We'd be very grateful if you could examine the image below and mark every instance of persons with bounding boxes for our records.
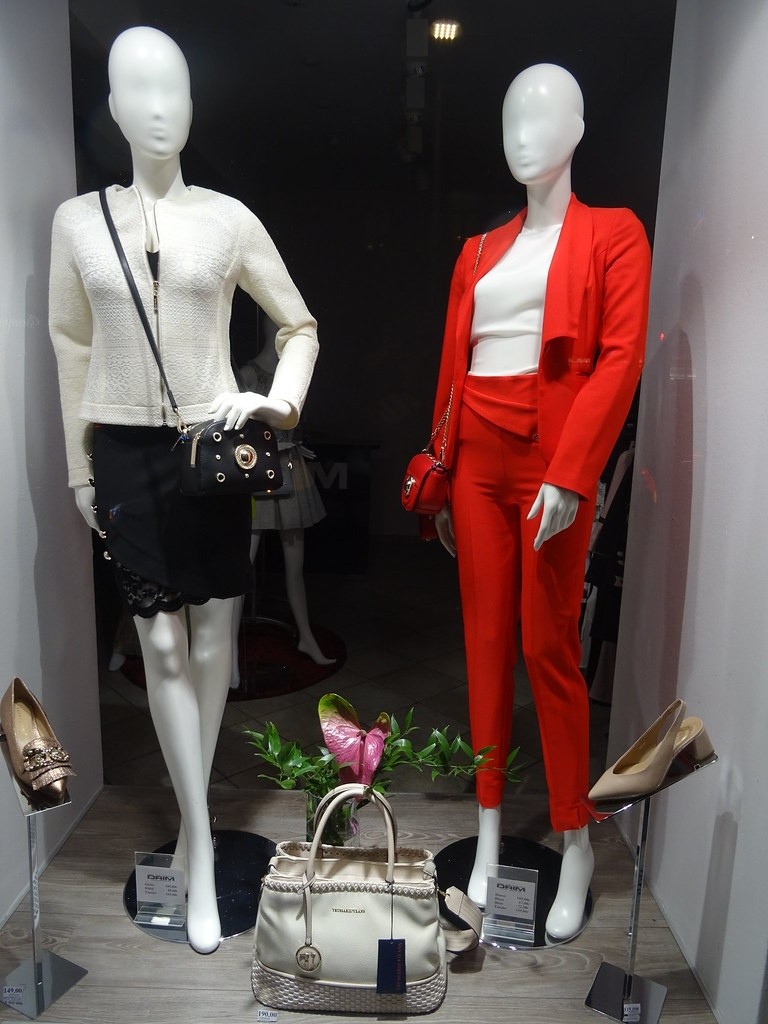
[226,313,337,688]
[48,25,322,954]
[432,64,650,939]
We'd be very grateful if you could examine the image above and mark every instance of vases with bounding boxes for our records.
[305,791,360,847]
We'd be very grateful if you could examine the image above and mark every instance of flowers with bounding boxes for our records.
[241,692,527,846]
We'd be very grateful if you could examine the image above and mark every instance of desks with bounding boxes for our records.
[259,436,382,576]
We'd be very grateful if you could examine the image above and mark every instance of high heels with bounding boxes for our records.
[589,695,714,801]
[0,677,78,809]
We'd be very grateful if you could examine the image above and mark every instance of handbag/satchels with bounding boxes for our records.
[398,234,488,520]
[94,188,287,507]
[249,780,447,1015]
